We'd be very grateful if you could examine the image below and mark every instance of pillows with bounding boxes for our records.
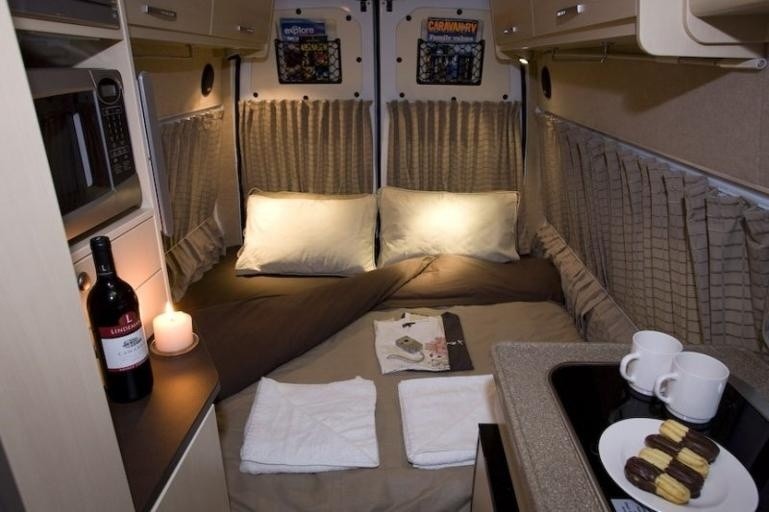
[376,185,521,269]
[235,190,379,278]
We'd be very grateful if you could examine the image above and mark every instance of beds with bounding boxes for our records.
[175,245,586,512]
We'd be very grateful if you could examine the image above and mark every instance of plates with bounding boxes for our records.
[597,417,758,511]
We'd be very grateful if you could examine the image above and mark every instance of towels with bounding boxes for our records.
[397,374,494,470]
[239,375,380,475]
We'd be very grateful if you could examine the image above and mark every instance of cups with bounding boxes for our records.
[620,329,730,424]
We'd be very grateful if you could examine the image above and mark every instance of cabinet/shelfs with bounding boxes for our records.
[74,216,168,387]
[470,424,519,512]
[125,0,273,50]
[490,0,639,52]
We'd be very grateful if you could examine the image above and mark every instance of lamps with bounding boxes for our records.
[153,301,194,353]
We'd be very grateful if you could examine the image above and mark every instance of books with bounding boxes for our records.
[424,14,480,84]
[275,15,332,84]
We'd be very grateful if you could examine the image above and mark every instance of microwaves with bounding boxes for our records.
[24,66,143,243]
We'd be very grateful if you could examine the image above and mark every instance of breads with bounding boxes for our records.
[626,418,720,505]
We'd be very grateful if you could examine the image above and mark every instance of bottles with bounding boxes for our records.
[83,233,155,401]
